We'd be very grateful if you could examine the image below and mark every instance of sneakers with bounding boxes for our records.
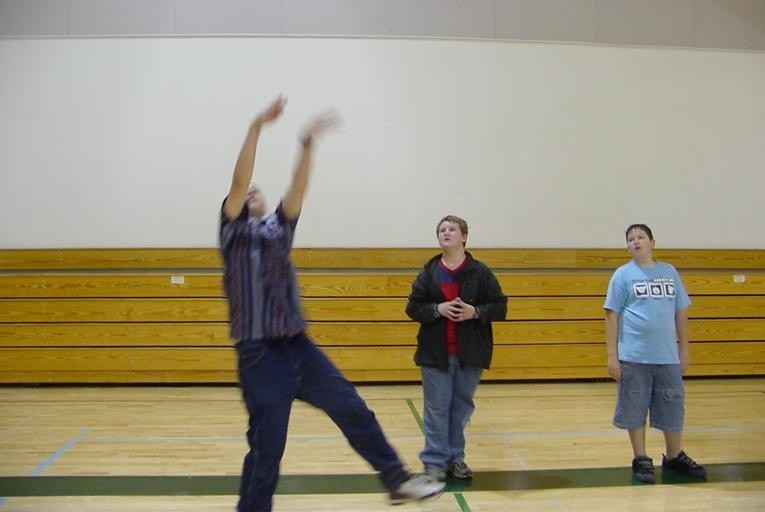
[389,475,446,506]
[662,450,707,478]
[449,462,473,479]
[632,455,656,483]
[424,464,447,481]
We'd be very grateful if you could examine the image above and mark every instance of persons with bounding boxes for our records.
[602,222,708,483]
[403,215,509,482]
[219,92,449,512]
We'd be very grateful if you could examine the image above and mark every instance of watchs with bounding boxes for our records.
[433,304,440,319]
[473,307,479,319]
